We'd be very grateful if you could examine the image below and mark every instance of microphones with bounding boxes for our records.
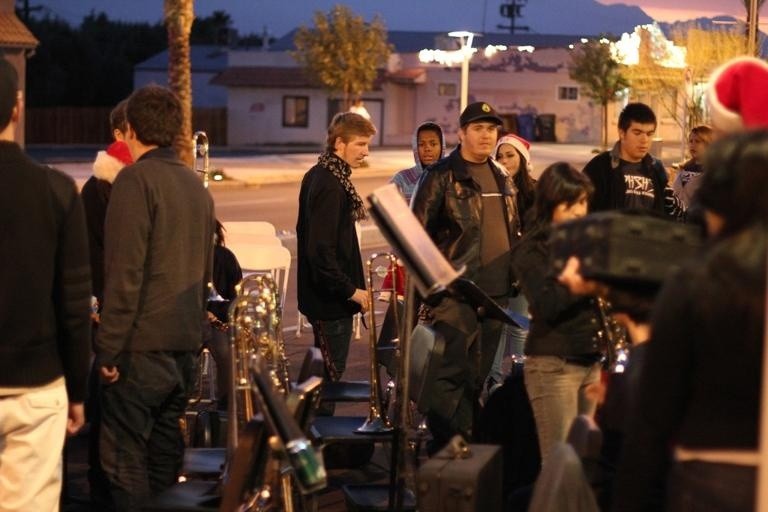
[252,362,331,495]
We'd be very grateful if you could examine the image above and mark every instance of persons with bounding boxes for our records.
[295,111,377,462]
[1,55,93,511]
[379,124,450,378]
[78,95,132,319]
[580,100,670,219]
[510,160,613,512]
[524,127,768,512]
[94,81,217,510]
[410,102,525,458]
[492,135,540,367]
[673,125,710,209]
[179,215,242,448]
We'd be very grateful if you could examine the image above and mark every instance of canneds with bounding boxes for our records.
[284,436,329,486]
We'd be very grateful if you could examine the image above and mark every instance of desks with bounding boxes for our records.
[221,221,291,316]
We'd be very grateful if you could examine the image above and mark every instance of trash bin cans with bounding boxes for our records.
[537,114,556,141]
[499,114,518,136]
[517,115,537,140]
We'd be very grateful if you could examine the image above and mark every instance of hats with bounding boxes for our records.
[494,133,533,172]
[704,56,768,134]
[460,101,502,126]
[91,141,134,182]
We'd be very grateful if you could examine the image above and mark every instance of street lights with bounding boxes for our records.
[448,29,481,117]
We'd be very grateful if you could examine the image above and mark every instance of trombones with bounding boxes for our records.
[354,251,404,434]
[231,273,294,512]
[191,131,210,406]
